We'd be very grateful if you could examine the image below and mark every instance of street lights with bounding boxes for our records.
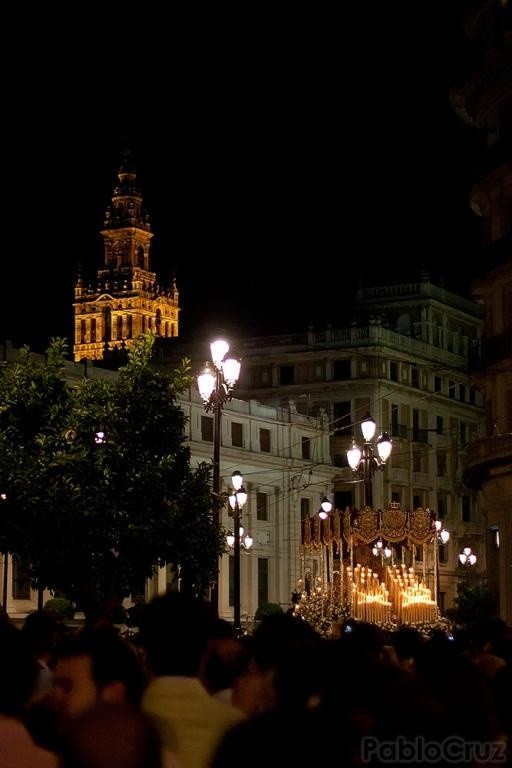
[459,546,477,586]
[226,524,254,551]
[195,333,244,615]
[228,470,249,631]
[344,410,394,506]
[428,511,450,610]
[372,539,392,570]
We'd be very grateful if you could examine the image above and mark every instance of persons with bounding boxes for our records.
[2,590,512,768]
